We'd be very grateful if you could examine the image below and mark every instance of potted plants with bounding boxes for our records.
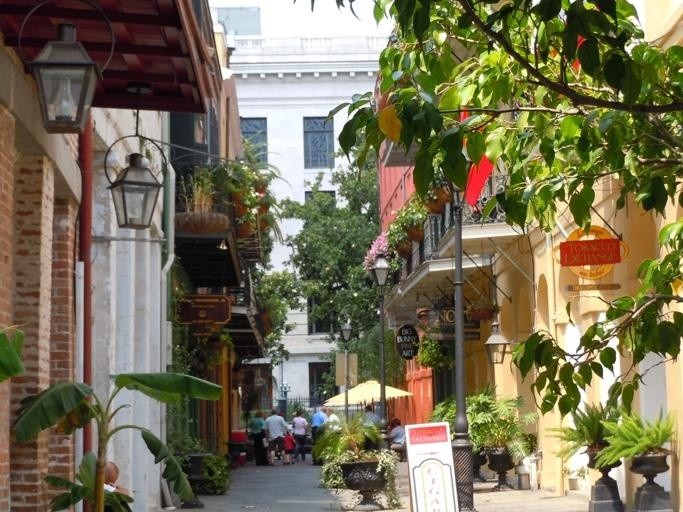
[427,384,539,494]
[215,134,292,245]
[319,447,404,511]
[545,397,682,510]
[385,180,453,258]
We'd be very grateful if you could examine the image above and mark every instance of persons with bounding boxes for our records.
[248,406,406,465]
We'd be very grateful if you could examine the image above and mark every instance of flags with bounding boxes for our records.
[459,106,495,207]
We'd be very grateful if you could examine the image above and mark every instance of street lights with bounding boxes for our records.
[341,323,353,424]
[367,253,392,449]
[280,381,291,421]
[438,152,474,511]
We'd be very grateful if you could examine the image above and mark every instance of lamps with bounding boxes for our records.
[13,2,116,138]
[102,86,167,231]
[486,320,529,366]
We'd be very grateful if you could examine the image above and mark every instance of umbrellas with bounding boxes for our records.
[323,379,412,413]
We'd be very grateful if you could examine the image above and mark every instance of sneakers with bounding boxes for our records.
[269,457,304,464]
